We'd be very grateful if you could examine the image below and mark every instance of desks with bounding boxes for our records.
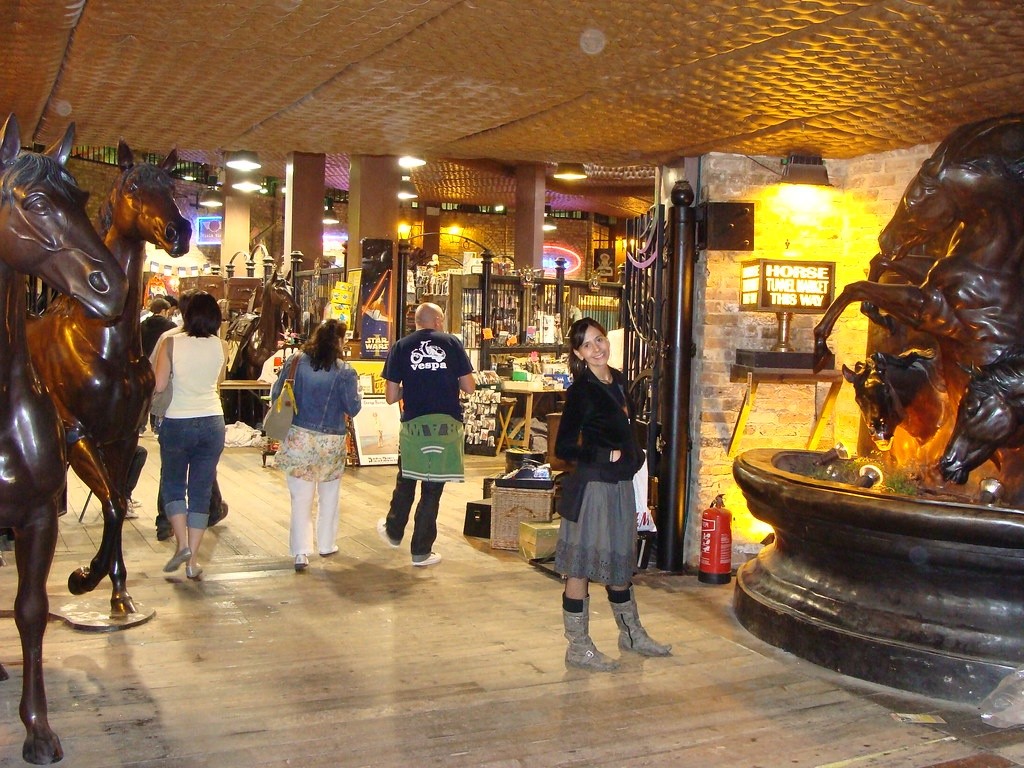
[497,402,517,454]
[218,380,271,468]
[502,388,568,449]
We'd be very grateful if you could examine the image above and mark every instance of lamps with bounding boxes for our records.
[232,171,262,192]
[226,150,261,171]
[813,442,849,469]
[398,178,418,199]
[323,198,340,225]
[856,463,884,488]
[777,158,834,187]
[978,476,1006,504]
[554,162,588,180]
[197,175,224,206]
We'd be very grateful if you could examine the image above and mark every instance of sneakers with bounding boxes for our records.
[412,552,442,566]
[378,517,400,549]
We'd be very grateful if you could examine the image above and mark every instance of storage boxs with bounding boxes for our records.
[464,498,491,538]
[518,521,562,560]
[326,281,354,329]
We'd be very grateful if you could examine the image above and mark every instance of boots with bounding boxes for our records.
[563,591,620,672]
[606,583,672,656]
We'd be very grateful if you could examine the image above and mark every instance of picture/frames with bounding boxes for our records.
[351,397,402,467]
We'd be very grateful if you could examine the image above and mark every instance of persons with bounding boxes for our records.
[271,319,362,570]
[376,302,476,566]
[140,288,228,577]
[554,317,672,671]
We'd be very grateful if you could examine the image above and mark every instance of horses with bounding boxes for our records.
[225,270,302,425]
[0,110,193,766]
[812,112,1024,510]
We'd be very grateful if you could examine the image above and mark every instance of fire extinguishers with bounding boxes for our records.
[696,494,734,584]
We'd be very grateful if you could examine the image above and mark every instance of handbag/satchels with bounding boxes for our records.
[148,371,174,418]
[557,477,587,522]
[262,378,298,443]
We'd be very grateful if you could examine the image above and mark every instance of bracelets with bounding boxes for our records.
[610,450,613,462]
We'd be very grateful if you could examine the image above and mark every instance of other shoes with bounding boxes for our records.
[123,498,141,518]
[294,553,309,570]
[320,545,338,556]
[156,521,175,541]
[162,546,201,578]
[208,503,228,526]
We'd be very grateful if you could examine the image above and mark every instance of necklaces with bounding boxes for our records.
[600,373,612,384]
